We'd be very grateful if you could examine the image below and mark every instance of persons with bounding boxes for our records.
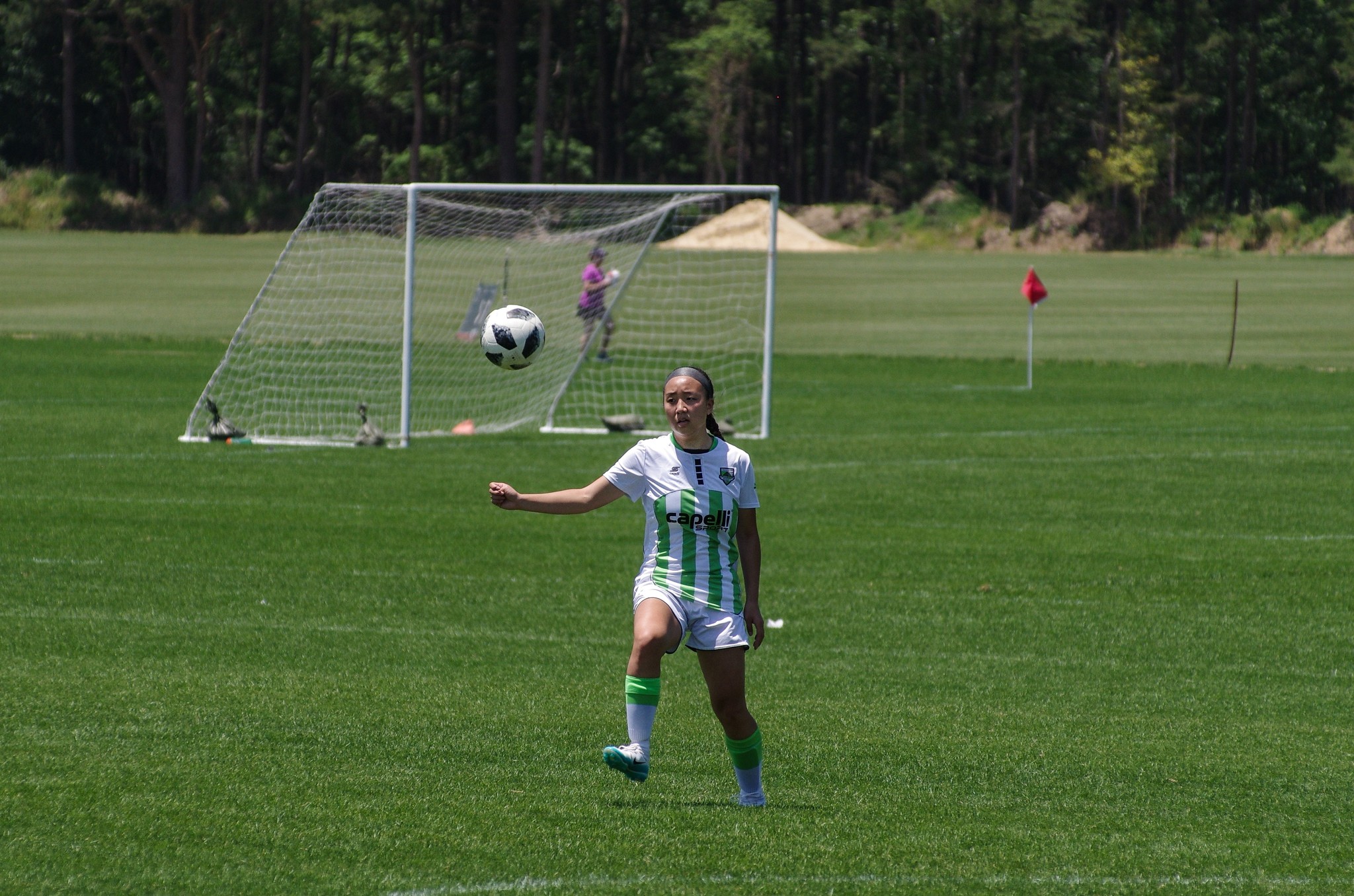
[489,366,764,808]
[576,246,620,362]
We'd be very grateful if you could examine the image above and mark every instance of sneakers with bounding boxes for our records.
[603,744,649,782]
[729,787,766,809]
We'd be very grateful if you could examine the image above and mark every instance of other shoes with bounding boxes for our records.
[595,354,611,364]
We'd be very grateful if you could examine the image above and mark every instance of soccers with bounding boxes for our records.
[605,269,621,285]
[480,304,545,370]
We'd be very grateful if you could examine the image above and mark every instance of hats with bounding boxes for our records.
[589,247,608,259]
